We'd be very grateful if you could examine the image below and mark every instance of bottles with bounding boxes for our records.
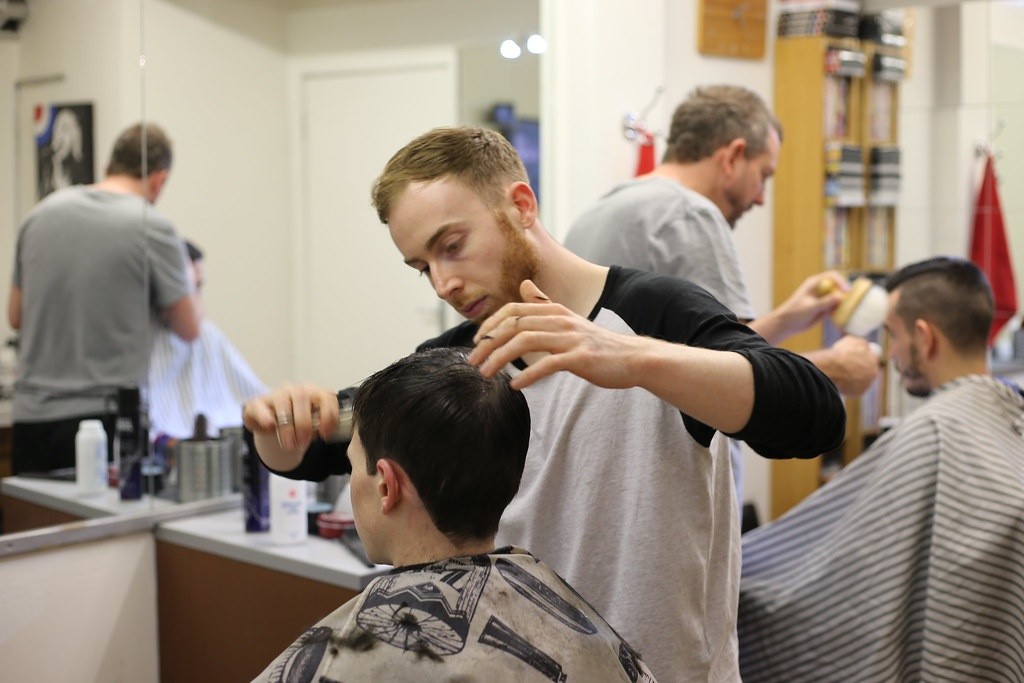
[76,420,107,495]
[268,470,309,545]
[241,444,270,529]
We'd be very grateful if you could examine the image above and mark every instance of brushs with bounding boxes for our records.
[818,277,892,338]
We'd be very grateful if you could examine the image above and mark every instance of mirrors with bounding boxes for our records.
[0,0,540,536]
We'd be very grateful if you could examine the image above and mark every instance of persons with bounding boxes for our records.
[9,122,197,475]
[250,346,655,683]
[560,84,1024,682]
[145,237,274,443]
[244,126,844,683]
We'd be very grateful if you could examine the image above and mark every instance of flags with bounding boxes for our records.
[966,147,1018,354]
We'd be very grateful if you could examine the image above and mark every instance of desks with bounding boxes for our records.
[155,508,396,683]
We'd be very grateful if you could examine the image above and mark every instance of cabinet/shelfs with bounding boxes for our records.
[770,0,913,521]
